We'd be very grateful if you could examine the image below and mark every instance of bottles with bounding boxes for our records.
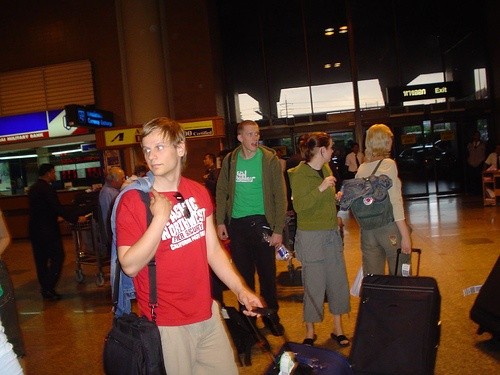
[277,244,290,259]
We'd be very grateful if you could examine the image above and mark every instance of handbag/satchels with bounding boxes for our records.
[350,190,395,231]
[75,191,99,212]
[282,218,297,251]
[104,312,167,375]
[350,266,364,298]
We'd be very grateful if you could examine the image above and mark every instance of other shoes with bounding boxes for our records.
[43,289,60,301]
[263,313,285,336]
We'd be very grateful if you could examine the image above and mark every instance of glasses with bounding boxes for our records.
[175,192,191,219]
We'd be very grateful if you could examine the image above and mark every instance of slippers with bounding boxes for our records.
[331,333,349,347]
[304,339,313,347]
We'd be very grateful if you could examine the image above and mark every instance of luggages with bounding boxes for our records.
[220,303,259,354]
[349,248,441,375]
[239,305,354,375]
[469,256,500,344]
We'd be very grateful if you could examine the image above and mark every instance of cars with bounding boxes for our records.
[398,139,458,181]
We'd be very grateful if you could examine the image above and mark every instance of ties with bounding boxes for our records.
[355,155,360,169]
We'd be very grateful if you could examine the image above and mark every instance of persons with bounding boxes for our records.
[0,214,27,357]
[113,116,264,375]
[135,166,147,178]
[289,132,353,349]
[466,131,487,197]
[353,123,413,279]
[345,142,365,177]
[100,165,126,241]
[202,151,220,193]
[27,164,90,300]
[483,144,500,177]
[215,120,289,337]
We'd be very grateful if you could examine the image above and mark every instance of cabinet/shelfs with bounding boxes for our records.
[482,164,500,207]
